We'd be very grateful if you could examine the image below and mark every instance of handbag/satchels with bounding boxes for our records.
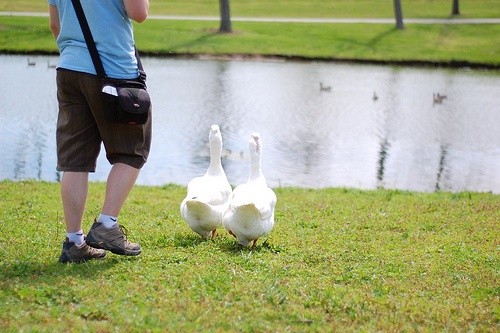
[98,77,150,124]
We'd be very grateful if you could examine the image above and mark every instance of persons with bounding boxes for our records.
[49,0,152,263]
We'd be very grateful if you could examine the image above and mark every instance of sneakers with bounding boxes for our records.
[59,236,106,263]
[84,217,142,258]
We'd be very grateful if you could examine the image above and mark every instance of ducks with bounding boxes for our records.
[180,124,277,250]
